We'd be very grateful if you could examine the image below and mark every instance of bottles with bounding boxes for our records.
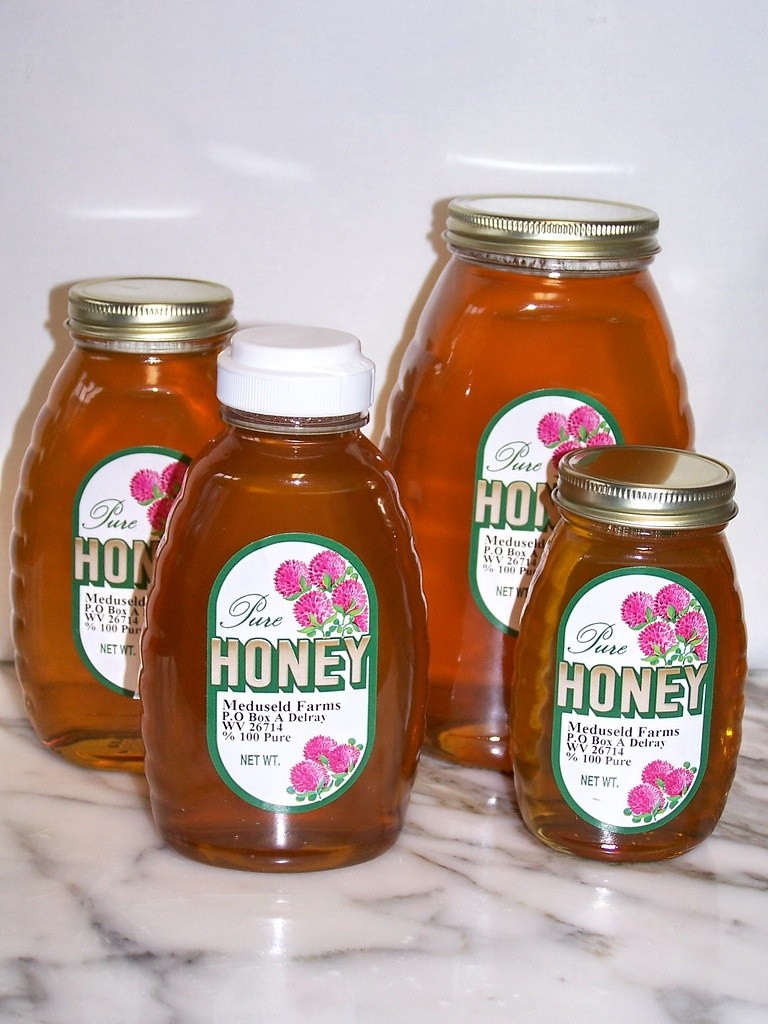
[15,275,236,768]
[140,324,428,874]
[381,193,693,768]
[506,444,746,864]
